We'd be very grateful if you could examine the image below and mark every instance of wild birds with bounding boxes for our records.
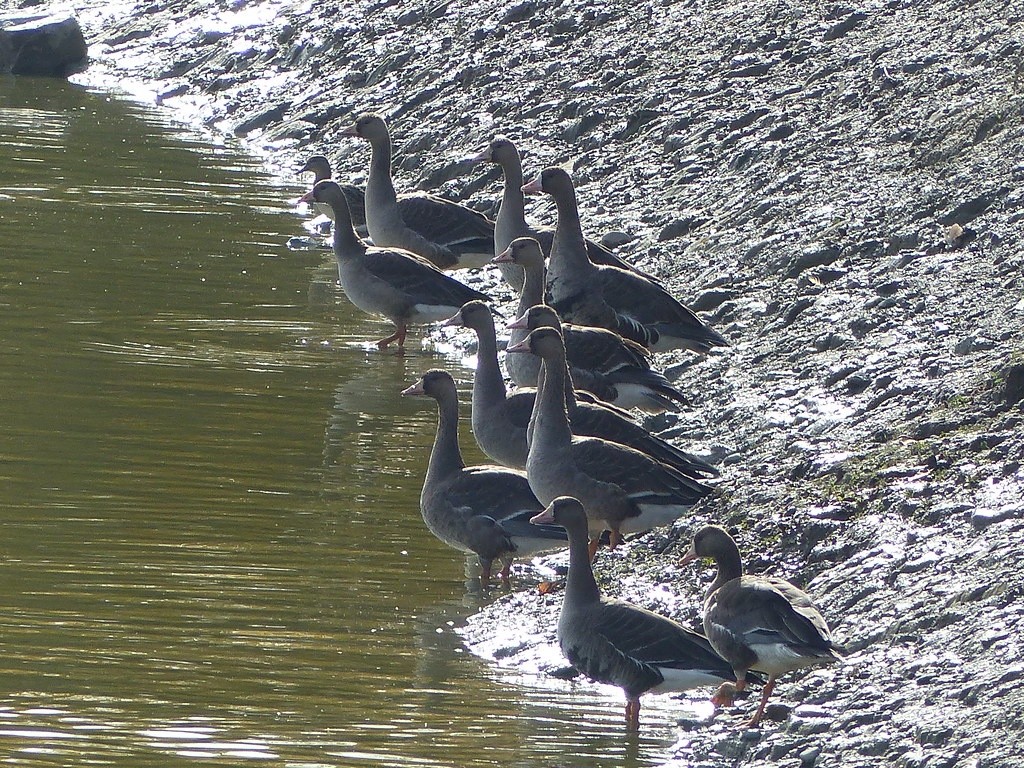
[679,524,848,730]
[471,137,669,295]
[438,300,637,470]
[504,327,715,564]
[489,236,691,416]
[528,495,768,727]
[504,304,723,480]
[403,368,609,574]
[520,165,733,355]
[295,179,509,347]
[337,112,496,273]
[291,152,370,240]
